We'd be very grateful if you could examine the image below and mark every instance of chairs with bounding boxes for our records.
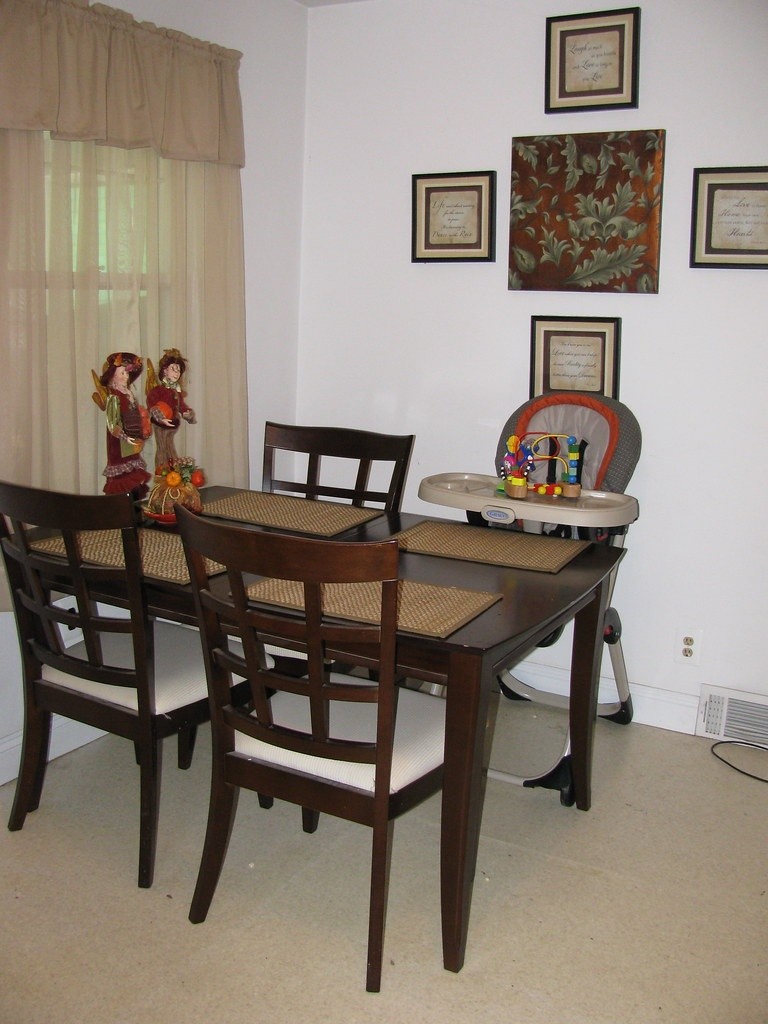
[0,480,283,888]
[262,422,416,685]
[418,392,642,805]
[173,501,448,993]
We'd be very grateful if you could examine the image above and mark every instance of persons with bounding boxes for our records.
[98,350,152,494]
[146,349,198,476]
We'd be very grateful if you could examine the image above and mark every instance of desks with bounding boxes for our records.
[7,486,628,972]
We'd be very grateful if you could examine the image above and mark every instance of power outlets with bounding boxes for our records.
[675,627,702,663]
[52,595,84,649]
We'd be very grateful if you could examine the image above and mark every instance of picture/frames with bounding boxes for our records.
[689,166,768,270]
[411,170,497,263]
[544,6,641,114]
[528,315,622,402]
